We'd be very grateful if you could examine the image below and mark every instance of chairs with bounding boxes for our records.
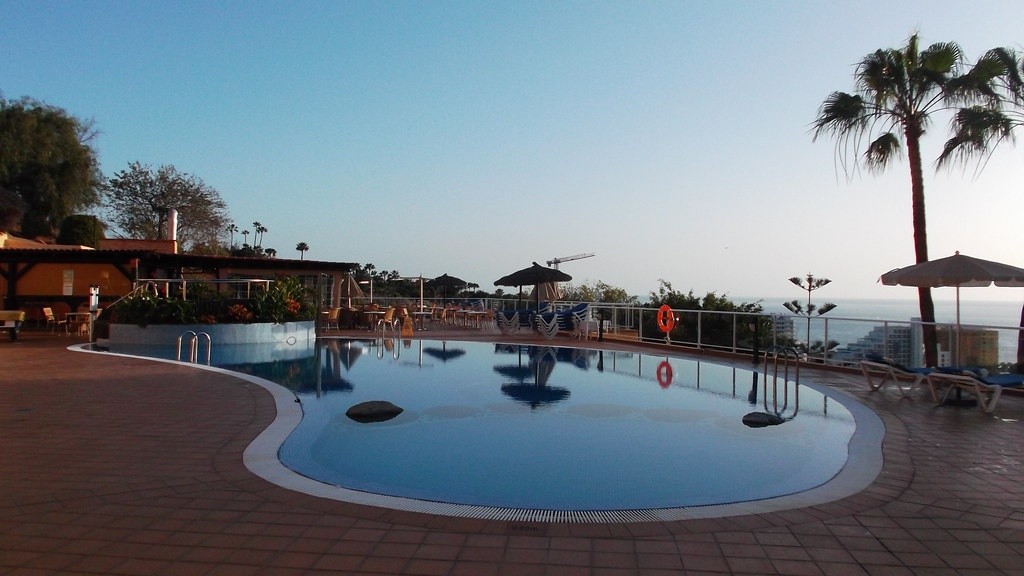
[319,302,495,334]
[496,301,592,340]
[858,359,1024,414]
[43,307,103,337]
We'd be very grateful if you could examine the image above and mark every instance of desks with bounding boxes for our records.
[363,312,385,333]
[65,313,90,337]
[413,311,432,331]
[458,309,474,329]
[468,311,488,330]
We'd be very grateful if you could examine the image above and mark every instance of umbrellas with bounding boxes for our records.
[877,251,1024,369]
[429,273,468,308]
[493,262,573,315]
[340,273,363,298]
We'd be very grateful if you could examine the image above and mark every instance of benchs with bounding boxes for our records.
[0,311,25,341]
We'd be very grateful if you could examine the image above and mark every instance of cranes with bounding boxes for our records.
[546,252,596,271]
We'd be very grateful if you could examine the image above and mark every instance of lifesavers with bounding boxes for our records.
[657,304,675,333]
[656,362,673,389]
[143,281,158,299]
[254,282,266,297]
